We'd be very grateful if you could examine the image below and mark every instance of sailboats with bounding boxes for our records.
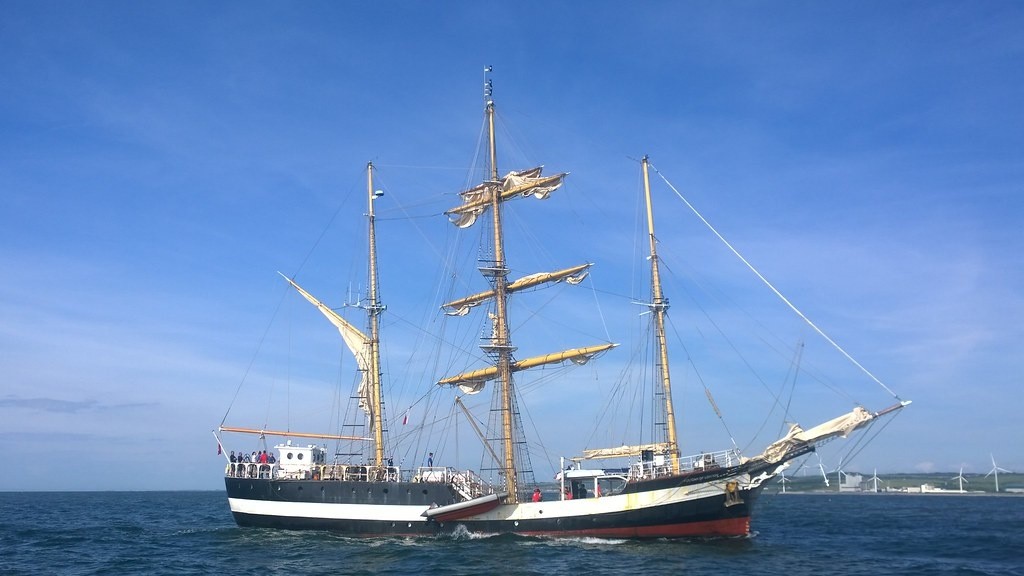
[210,63,912,540]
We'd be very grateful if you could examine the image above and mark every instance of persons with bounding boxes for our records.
[598,482,601,498]
[229,450,275,479]
[388,456,394,473]
[532,486,542,502]
[578,484,587,499]
[559,487,568,500]
[428,453,434,471]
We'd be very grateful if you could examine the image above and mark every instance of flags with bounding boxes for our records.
[402,409,410,426]
